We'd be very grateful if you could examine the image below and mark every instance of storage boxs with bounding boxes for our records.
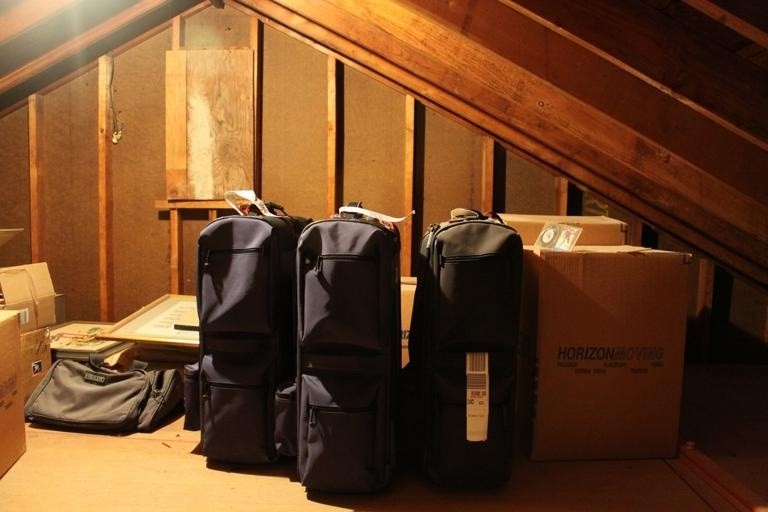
[0,261,62,480]
[499,209,691,459]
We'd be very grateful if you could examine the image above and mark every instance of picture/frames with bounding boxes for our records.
[48,293,205,362]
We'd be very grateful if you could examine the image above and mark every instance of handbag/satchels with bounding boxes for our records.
[194,206,313,469]
[292,200,408,502]
[22,357,186,435]
[407,214,525,500]
